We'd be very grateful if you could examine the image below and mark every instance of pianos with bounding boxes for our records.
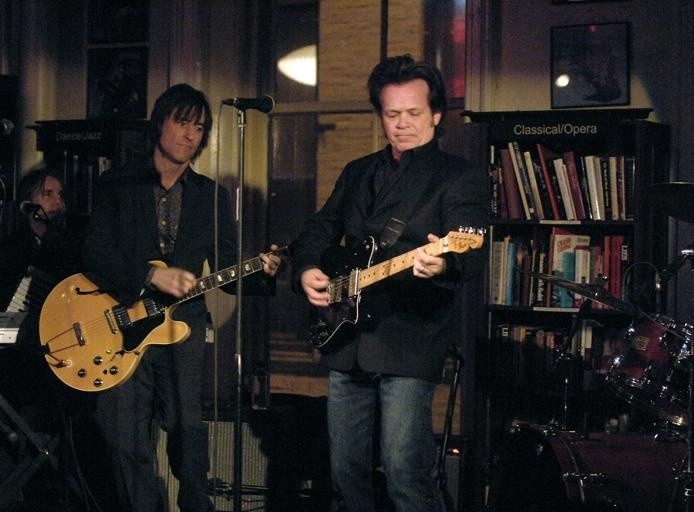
[1,311,38,345]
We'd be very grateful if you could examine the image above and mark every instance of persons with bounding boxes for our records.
[1,168,101,511]
[292,52,495,510]
[77,81,281,510]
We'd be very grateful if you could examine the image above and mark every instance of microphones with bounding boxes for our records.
[223,93,277,115]
[18,198,43,218]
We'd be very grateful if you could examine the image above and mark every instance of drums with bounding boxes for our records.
[502,421,691,512]
[602,310,693,428]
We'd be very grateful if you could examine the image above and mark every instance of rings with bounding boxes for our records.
[417,266,425,272]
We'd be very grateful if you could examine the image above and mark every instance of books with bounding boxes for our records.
[483,138,630,371]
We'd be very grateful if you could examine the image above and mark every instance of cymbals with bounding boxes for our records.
[512,267,644,320]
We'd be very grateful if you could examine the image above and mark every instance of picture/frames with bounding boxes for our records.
[551,21,630,109]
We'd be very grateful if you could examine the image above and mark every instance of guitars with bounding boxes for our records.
[39,238,293,394]
[311,224,487,354]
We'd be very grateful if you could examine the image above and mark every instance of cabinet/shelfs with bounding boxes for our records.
[461,107,673,424]
